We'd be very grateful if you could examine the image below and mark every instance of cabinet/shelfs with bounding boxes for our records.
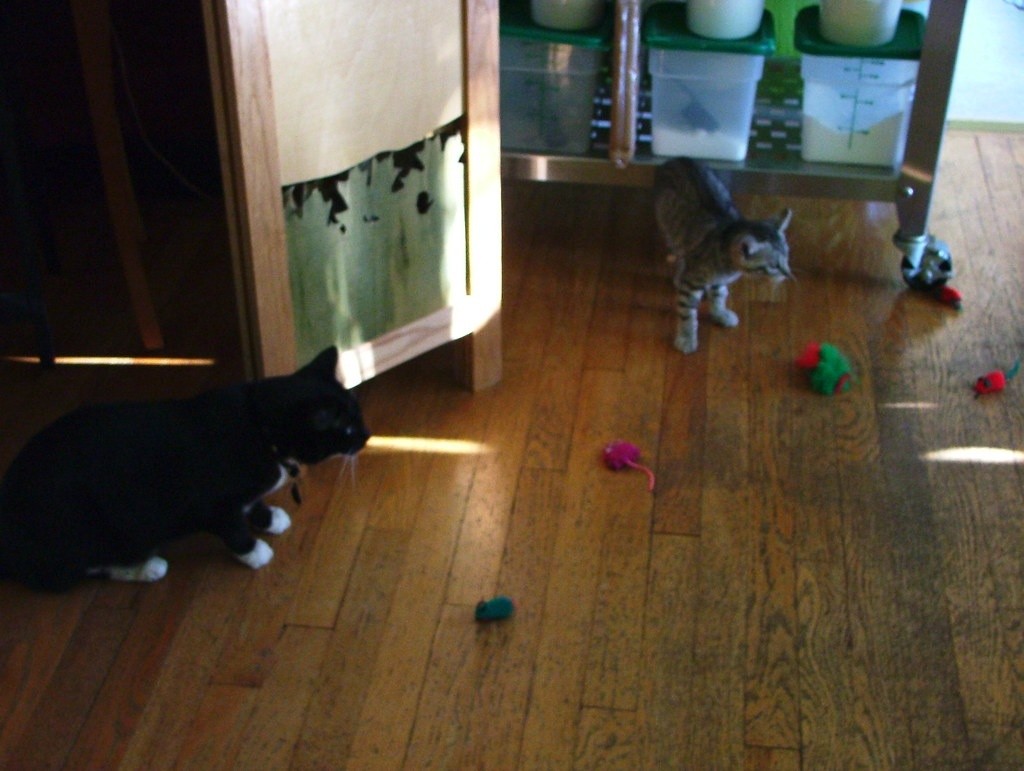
[500,0,968,290]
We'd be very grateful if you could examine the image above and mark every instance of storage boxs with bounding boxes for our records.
[642,1,776,160]
[794,4,927,167]
[499,30,605,153]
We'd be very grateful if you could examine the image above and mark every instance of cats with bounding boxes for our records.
[649,155,792,355]
[0,344,373,593]
